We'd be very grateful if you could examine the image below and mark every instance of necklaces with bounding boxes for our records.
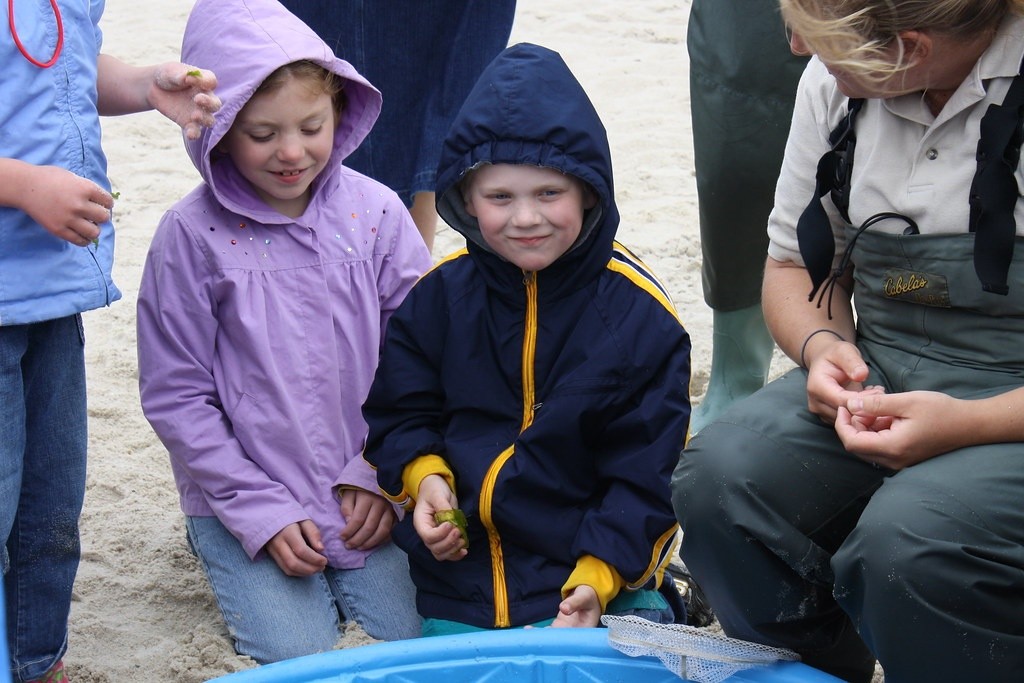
[8,0,63,68]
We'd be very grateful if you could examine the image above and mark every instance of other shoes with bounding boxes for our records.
[664,564,714,627]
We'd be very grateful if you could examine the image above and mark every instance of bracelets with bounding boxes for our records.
[802,329,844,371]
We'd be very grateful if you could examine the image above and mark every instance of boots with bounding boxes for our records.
[689,305,776,436]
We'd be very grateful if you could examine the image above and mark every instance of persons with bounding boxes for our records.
[275,0,517,256]
[137,1,435,667]
[361,43,694,635]
[0,0,222,683]
[687,0,811,437]
[673,0,1024,683]
